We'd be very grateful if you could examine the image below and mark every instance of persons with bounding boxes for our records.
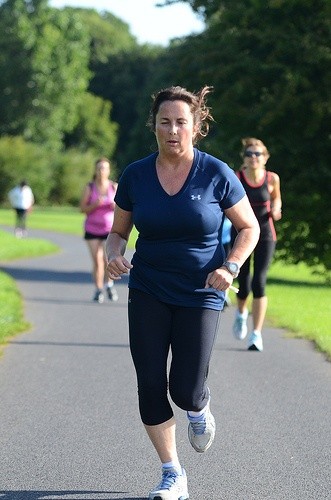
[106,85,260,500]
[8,180,34,238]
[80,159,119,303]
[233,137,282,352]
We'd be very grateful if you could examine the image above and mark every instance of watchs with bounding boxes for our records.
[222,262,239,278]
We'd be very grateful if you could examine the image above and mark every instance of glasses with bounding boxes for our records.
[244,150,264,157]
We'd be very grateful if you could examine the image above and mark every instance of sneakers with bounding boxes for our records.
[249,333,263,352]
[148,468,188,500]
[233,307,249,340]
[187,389,215,453]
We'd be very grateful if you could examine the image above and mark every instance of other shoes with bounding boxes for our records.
[106,285,119,301]
[94,290,102,303]
